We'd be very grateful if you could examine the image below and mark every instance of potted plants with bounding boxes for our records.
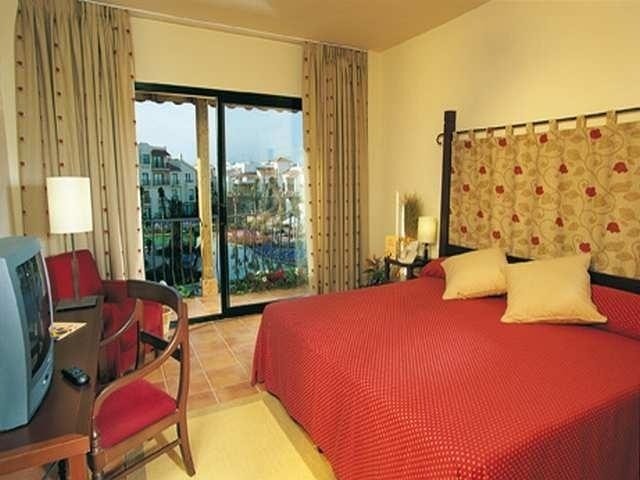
[402,192,420,256]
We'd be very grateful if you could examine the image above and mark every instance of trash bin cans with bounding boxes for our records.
[163,307,175,341]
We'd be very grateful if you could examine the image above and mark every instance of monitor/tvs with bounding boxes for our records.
[0,237,54,432]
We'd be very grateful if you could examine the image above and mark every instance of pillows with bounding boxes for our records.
[421,260,448,280]
[440,248,509,300]
[589,285,640,341]
[500,252,608,328]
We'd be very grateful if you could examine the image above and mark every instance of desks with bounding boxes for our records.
[0,294,105,480]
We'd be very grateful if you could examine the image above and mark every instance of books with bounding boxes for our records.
[52,321,86,341]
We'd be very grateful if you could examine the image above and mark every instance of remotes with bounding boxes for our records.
[61,365,91,386]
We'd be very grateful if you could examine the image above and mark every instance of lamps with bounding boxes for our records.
[417,216,437,262]
[45,175,98,312]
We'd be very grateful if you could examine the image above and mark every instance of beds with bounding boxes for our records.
[249,108,640,480]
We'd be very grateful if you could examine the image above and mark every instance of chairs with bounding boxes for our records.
[85,277,196,480]
[44,249,162,382]
